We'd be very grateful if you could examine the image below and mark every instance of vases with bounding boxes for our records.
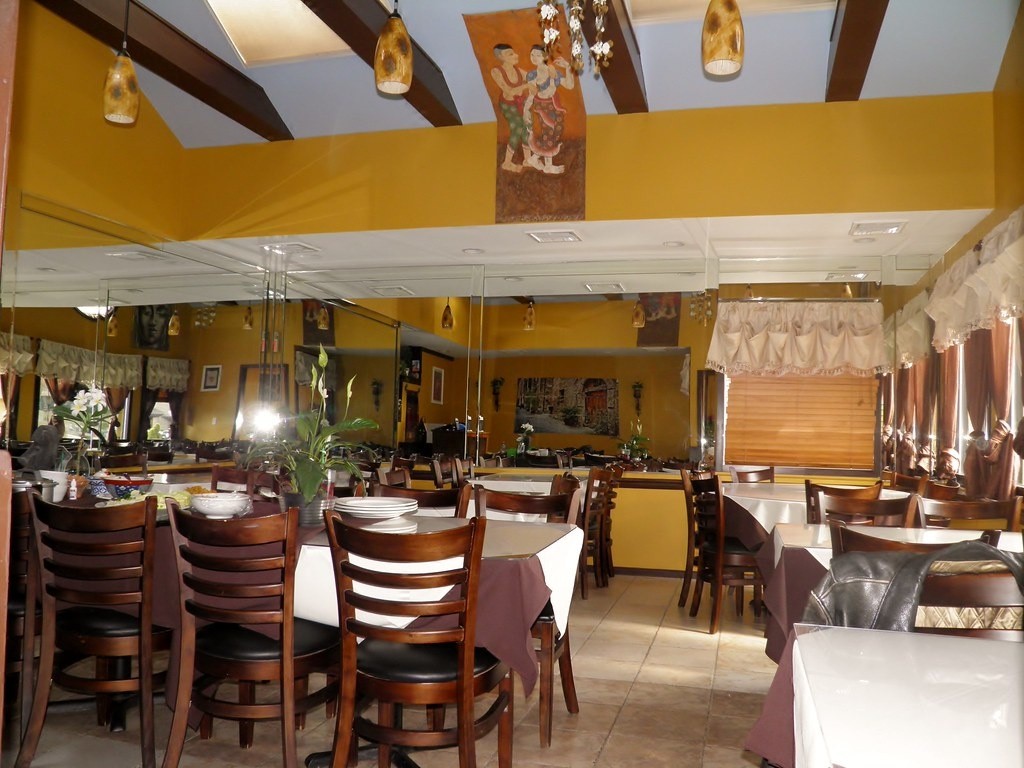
[64,446,93,499]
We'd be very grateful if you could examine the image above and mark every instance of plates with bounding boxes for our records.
[359,516,418,535]
[334,496,419,520]
[94,500,191,511]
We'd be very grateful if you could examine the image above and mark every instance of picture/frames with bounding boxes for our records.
[430,366,445,404]
[199,364,222,391]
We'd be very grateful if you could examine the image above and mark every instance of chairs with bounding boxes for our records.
[322,509,514,768]
[13,488,175,768]
[473,483,580,750]
[676,466,735,608]
[192,465,281,737]
[381,444,693,601]
[241,470,338,732]
[159,496,344,767]
[726,464,775,597]
[7,437,312,475]
[357,478,471,732]
[689,474,768,635]
[775,470,1024,645]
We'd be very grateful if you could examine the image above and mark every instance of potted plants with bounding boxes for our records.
[489,376,504,411]
[246,344,379,529]
[612,416,653,463]
[631,380,644,416]
[368,377,382,412]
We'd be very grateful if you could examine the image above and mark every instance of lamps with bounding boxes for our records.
[688,290,713,325]
[700,0,744,76]
[241,299,255,330]
[317,300,330,330]
[103,0,140,125]
[536,0,614,80]
[521,295,536,332]
[743,283,755,300]
[107,305,119,338]
[441,296,455,331]
[167,304,181,336]
[838,282,854,301]
[373,0,414,95]
[630,294,645,329]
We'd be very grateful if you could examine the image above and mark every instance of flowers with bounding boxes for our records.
[53,387,122,477]
[519,421,535,445]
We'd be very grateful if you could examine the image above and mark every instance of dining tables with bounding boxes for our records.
[43,491,584,747]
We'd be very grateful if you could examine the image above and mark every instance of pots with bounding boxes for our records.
[12,469,60,503]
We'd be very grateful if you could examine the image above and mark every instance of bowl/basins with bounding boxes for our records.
[190,492,250,519]
[87,477,106,496]
[102,476,153,499]
[526,449,548,456]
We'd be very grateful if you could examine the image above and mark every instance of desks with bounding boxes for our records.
[700,481,915,618]
[791,621,1024,767]
